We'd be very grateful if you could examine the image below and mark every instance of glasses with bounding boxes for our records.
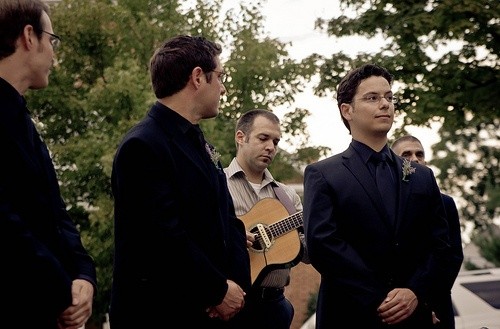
[34,27,62,48]
[348,93,398,105]
[201,69,228,84]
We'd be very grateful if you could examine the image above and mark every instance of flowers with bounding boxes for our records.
[402,159,415,183]
[206,142,224,168]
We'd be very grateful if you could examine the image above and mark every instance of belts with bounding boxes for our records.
[255,286,284,299]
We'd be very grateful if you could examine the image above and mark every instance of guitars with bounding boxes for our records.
[239,198,305,288]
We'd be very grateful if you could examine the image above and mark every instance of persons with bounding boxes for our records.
[389,135,464,329]
[303,63,450,329]
[108,34,253,329]
[0,0,103,329]
[222,108,303,329]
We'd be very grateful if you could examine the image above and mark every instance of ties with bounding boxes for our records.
[375,152,397,227]
